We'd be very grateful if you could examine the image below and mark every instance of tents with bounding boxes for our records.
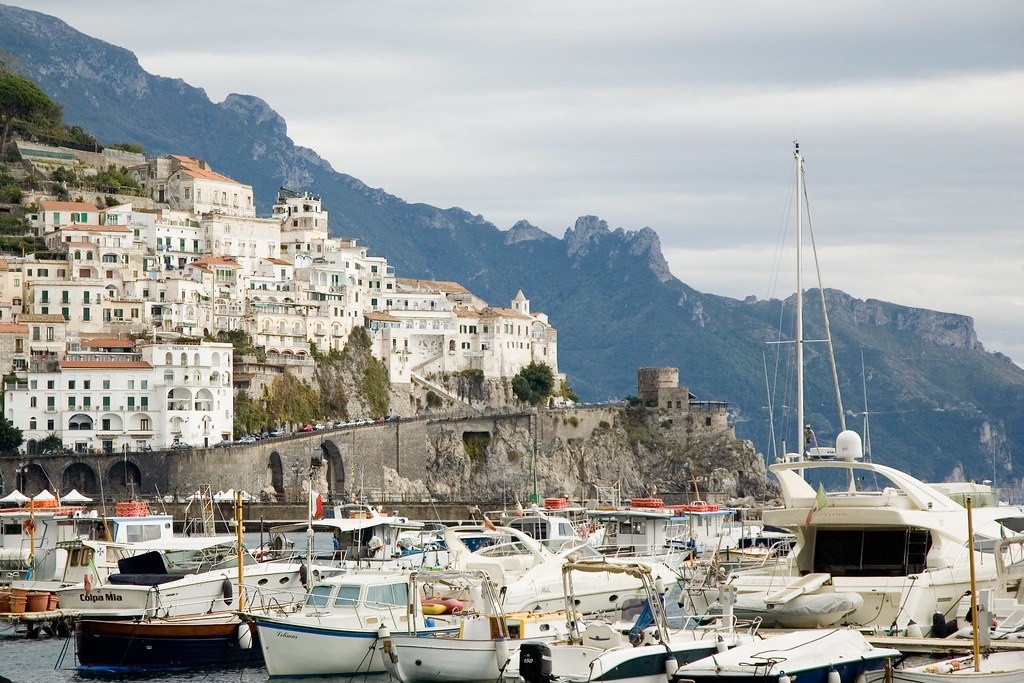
[186,488,257,503]
[0,488,94,510]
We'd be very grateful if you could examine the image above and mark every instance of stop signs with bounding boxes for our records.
[15,464,28,476]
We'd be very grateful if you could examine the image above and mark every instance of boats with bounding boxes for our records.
[0,139,1024,683]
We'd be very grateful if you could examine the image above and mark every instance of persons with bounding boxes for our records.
[172,486,179,504]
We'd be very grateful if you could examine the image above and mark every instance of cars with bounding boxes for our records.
[171,442,194,450]
[298,415,401,432]
[233,428,283,444]
[214,440,233,449]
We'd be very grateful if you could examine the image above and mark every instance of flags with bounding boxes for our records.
[311,489,324,519]
[804,484,829,529]
[83,559,99,596]
